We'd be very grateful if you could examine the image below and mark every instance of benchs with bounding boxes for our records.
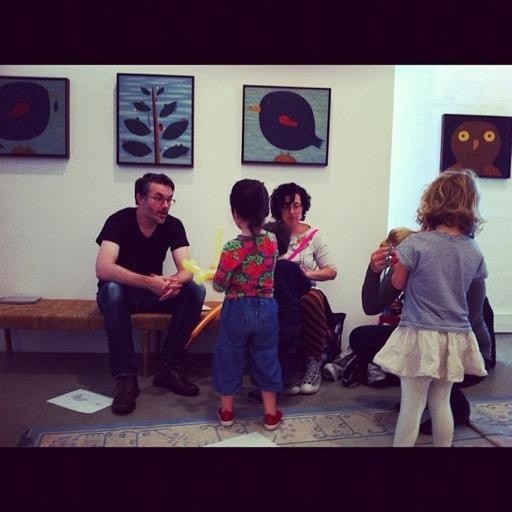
[1,295,226,378]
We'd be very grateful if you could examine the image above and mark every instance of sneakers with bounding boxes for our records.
[110,374,139,415]
[157,367,199,397]
[216,357,322,431]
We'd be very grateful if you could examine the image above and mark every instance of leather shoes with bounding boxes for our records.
[419,392,471,435]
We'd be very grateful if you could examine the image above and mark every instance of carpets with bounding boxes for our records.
[16,392,512,454]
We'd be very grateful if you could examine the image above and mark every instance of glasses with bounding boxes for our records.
[145,193,177,207]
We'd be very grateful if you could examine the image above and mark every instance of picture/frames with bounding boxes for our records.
[0,73,73,160]
[241,83,333,167]
[113,72,196,168]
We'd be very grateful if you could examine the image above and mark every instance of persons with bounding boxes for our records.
[212,179,282,431]
[96,174,205,415]
[248,221,312,404]
[349,227,496,434]
[371,172,490,448]
[268,183,337,394]
